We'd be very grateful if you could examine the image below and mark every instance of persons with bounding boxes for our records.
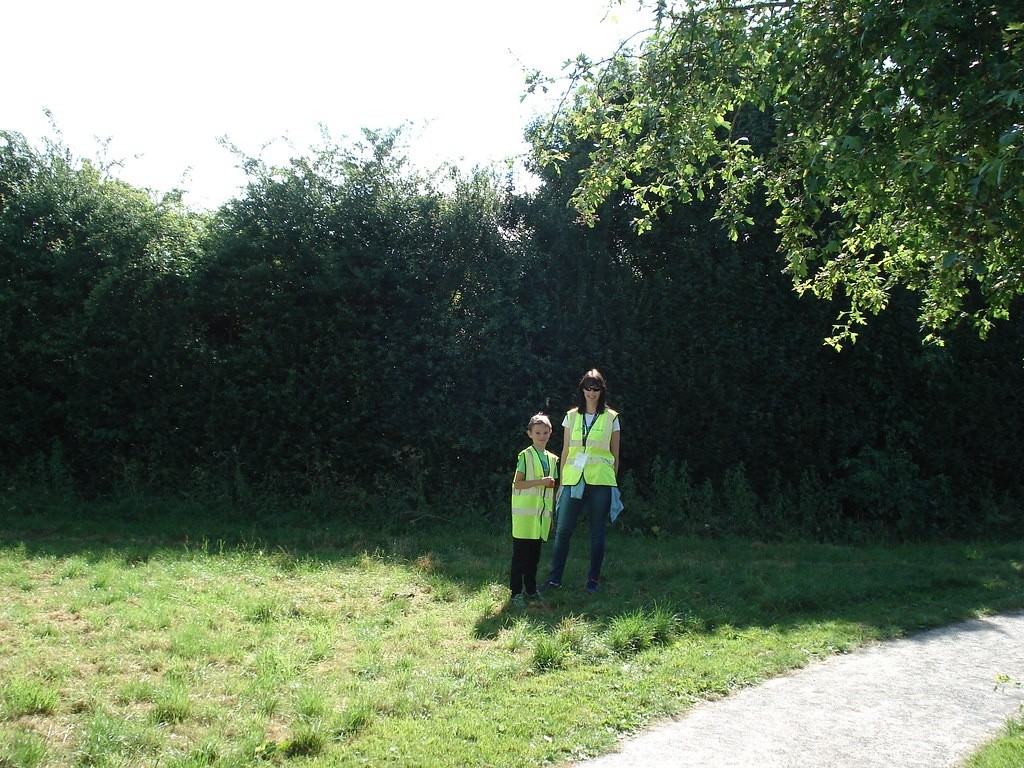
[536,369,621,595]
[511,412,559,603]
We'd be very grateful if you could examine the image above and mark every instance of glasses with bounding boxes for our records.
[584,386,601,392]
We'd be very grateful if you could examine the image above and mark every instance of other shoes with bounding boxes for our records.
[586,576,599,593]
[524,590,544,609]
[511,594,527,609]
[543,575,563,590]
